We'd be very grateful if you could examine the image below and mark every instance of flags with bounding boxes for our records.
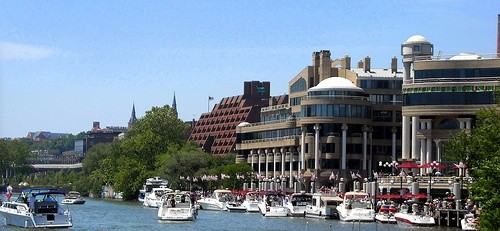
[209,97,214,100]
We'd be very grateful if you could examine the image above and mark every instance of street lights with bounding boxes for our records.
[431,161,439,175]
[399,172,404,188]
[379,161,399,169]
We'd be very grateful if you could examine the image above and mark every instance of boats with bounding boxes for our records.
[226,201,247,213]
[61,191,86,204]
[197,189,236,211]
[138,177,173,203]
[157,189,199,224]
[0,185,74,230]
[143,187,173,208]
[242,190,436,226]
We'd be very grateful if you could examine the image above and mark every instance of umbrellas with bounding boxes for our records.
[397,161,420,175]
[420,161,447,176]
[370,193,428,206]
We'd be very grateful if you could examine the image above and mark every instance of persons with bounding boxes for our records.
[29,193,35,213]
[44,195,52,202]
[181,195,185,203]
[234,194,246,203]
[170,197,176,208]
